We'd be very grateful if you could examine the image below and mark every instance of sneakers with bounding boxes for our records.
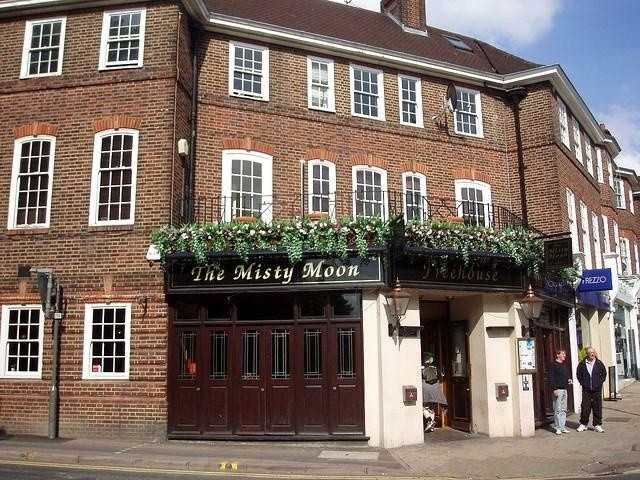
[563,430,571,434]
[556,431,562,435]
[425,420,437,433]
[577,424,588,432]
[594,425,605,433]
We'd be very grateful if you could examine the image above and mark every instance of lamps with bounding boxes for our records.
[519,284,545,339]
[383,280,412,350]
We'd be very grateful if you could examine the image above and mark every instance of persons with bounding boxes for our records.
[421,352,450,433]
[547,349,574,435]
[576,347,607,433]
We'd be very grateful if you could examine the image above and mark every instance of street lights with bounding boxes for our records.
[29,266,64,439]
